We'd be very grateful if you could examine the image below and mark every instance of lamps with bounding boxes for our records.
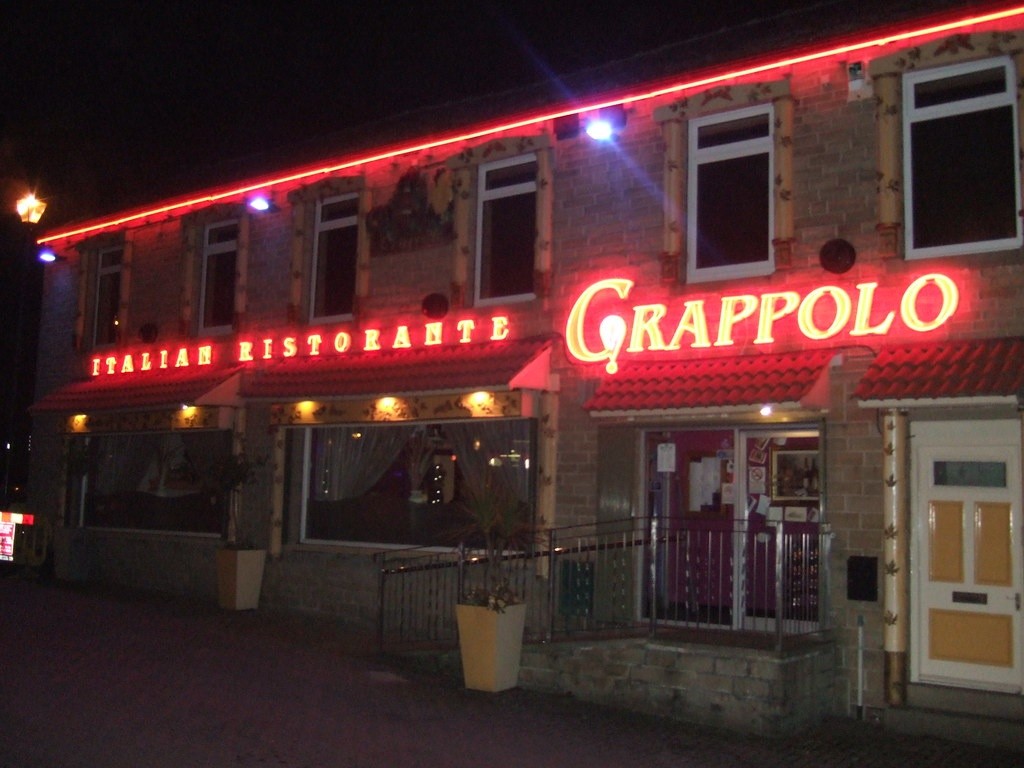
[40,250,67,265]
[846,61,872,104]
[248,195,280,215]
[656,433,675,477]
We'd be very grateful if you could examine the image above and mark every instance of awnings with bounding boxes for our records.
[29,336,551,416]
[584,335,830,419]
[851,332,1023,405]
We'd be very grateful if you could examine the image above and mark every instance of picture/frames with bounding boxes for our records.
[768,445,821,505]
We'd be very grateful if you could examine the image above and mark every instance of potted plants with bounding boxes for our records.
[435,454,560,694]
[214,535,265,609]
